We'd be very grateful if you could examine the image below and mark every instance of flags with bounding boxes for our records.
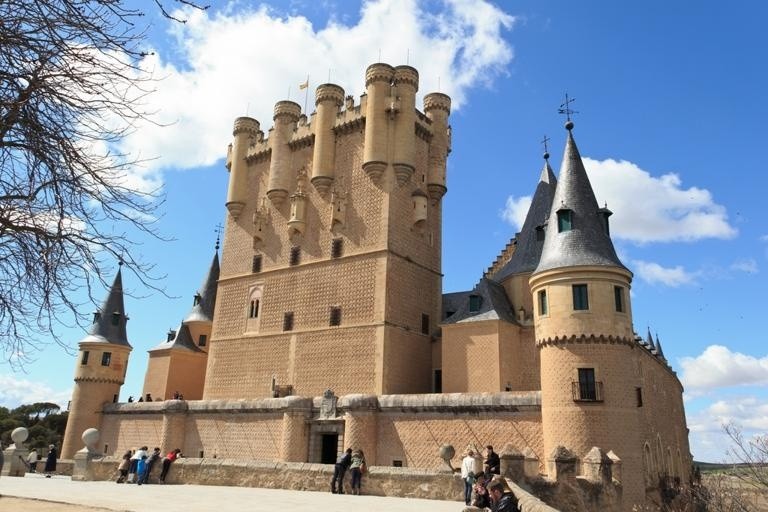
[299,83,308,89]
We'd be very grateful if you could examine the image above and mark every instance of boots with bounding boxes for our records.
[351,487,360,495]
[126,473,135,483]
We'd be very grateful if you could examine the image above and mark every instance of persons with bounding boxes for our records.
[117,446,182,485]
[331,448,367,495]
[45,444,57,478]
[128,396,133,402]
[27,448,38,473]
[174,390,183,399]
[139,397,143,402]
[273,384,280,397]
[461,445,518,512]
[506,381,511,391]
[146,393,152,401]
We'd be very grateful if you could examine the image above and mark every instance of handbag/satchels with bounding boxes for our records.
[466,476,474,485]
[359,462,367,474]
